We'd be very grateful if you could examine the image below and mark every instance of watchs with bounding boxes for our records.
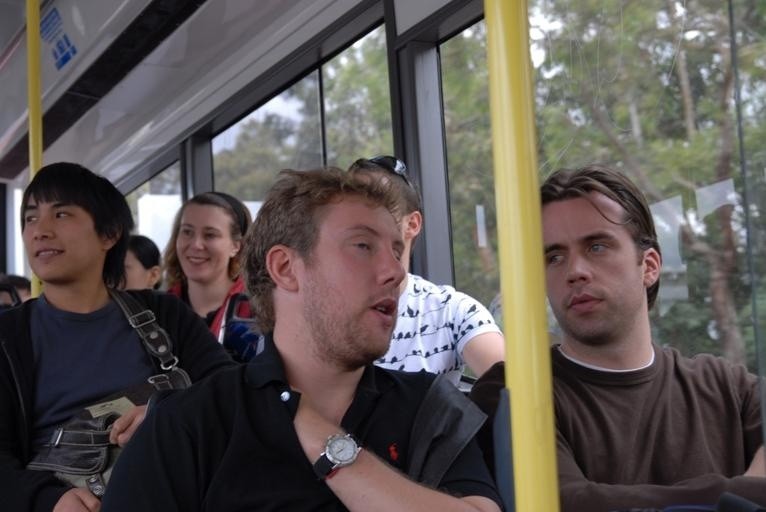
[311,432,363,484]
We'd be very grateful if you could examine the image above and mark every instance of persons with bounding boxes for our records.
[541,167,766,511]
[347,155,506,390]
[124,236,165,292]
[99,166,505,512]
[167,191,265,372]
[0,272,31,306]
[0,162,236,512]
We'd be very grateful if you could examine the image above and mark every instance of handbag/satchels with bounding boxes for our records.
[0,288,193,504]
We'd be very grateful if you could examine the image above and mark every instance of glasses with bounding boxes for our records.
[368,154,413,189]
